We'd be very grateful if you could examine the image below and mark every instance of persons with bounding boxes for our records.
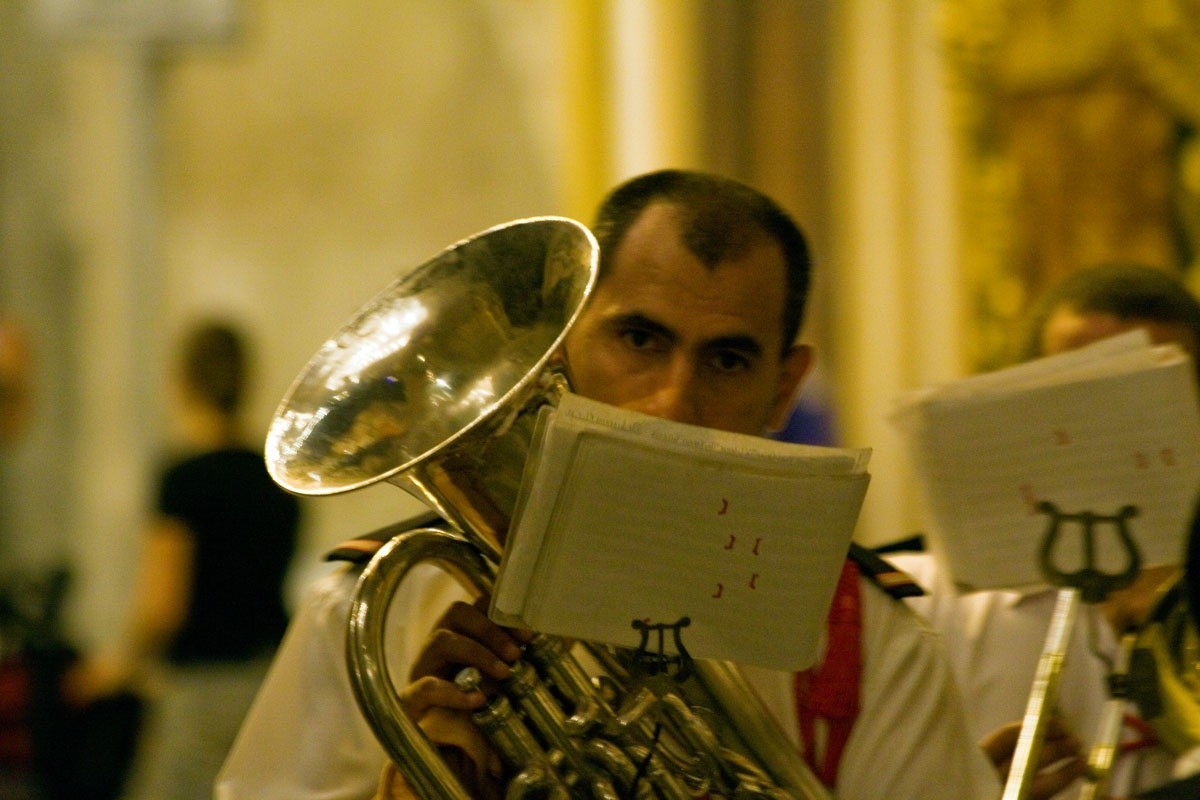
[0,306,157,800]
[214,171,1005,800]
[69,315,309,800]
[778,397,842,446]
[909,259,1200,800]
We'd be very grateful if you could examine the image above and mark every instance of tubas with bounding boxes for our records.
[265,216,839,799]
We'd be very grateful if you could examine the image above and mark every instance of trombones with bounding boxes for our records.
[1000,552,1198,800]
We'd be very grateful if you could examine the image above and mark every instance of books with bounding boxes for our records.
[491,390,872,673]
[887,331,1200,590]
